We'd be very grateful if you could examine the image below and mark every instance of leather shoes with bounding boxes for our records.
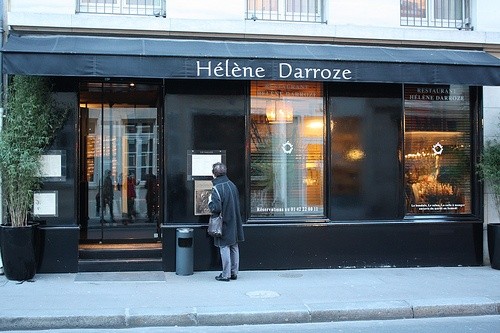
[215,276,229,281]
[220,273,237,280]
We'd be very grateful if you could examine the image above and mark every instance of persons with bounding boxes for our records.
[100,171,117,222]
[207,162,245,281]
[143,174,158,219]
[126,170,137,219]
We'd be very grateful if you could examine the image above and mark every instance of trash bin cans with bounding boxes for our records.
[175,228,194,275]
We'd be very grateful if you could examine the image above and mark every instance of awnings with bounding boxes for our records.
[0,34,500,87]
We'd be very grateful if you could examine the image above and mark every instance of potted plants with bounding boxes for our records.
[0,72,73,280]
[476,138,500,270]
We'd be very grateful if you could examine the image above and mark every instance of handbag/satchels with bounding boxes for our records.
[208,216,222,238]
